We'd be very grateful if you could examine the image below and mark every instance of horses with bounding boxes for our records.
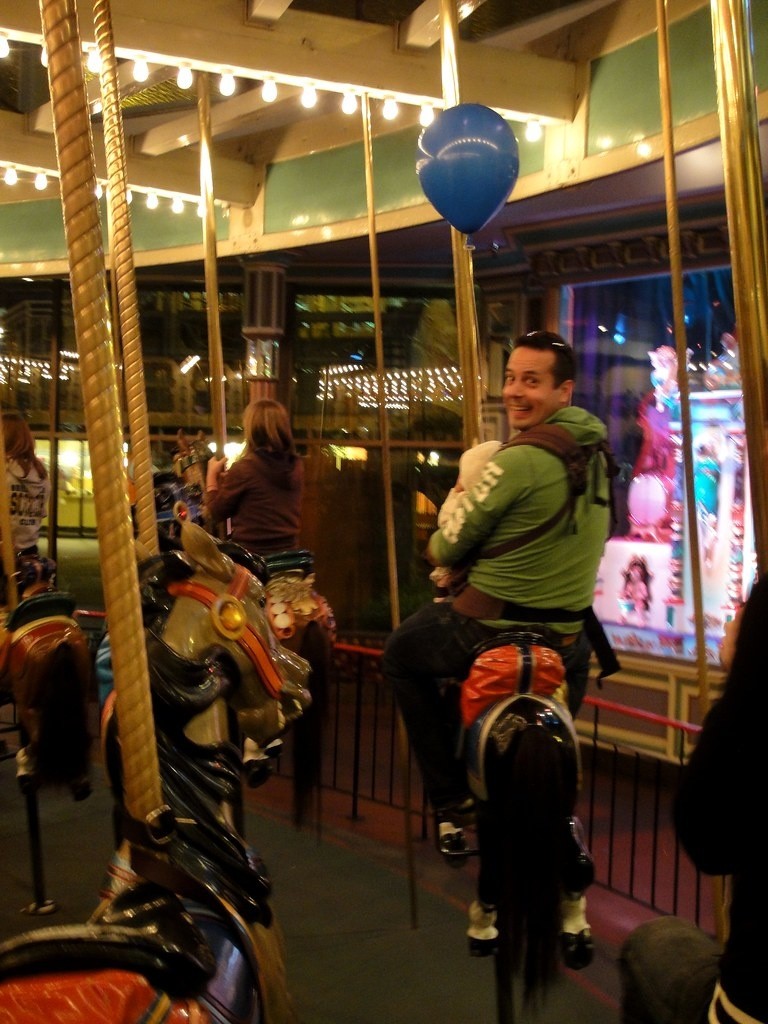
[1,514,596,1024]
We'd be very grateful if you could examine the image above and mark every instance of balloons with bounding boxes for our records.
[416,102,520,251]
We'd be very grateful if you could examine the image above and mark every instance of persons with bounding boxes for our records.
[204,400,302,555]
[0,413,50,558]
[607,572,768,1024]
[379,329,611,828]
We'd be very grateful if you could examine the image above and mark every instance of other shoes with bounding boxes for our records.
[449,798,476,815]
